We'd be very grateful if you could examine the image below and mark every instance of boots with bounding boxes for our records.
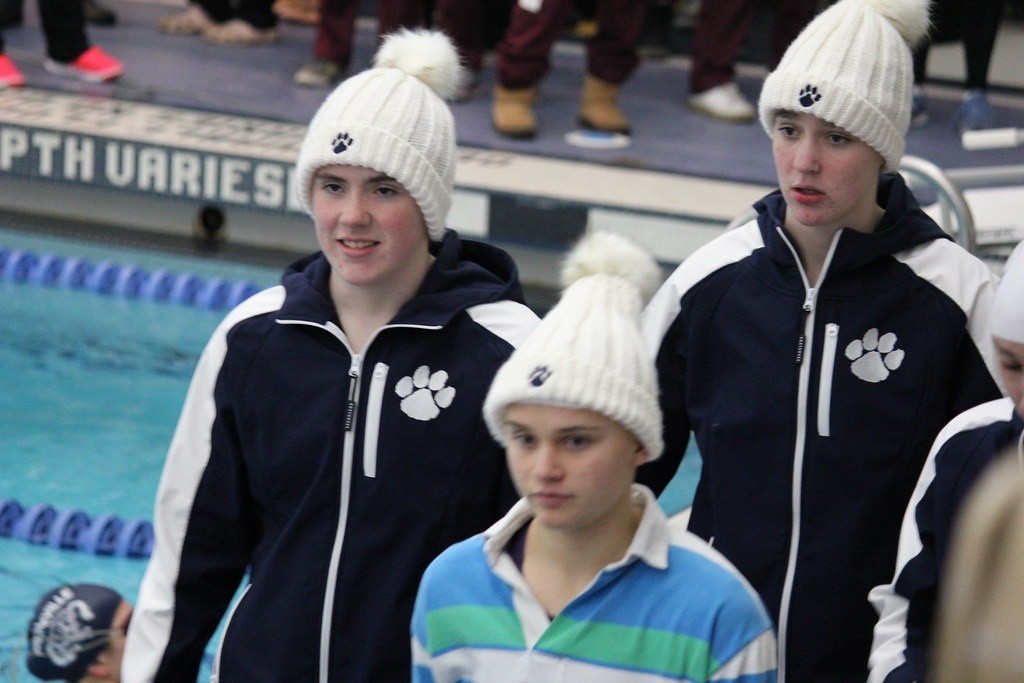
[578,75,631,138]
[492,84,537,134]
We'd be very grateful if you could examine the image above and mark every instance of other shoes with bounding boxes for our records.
[85,0,117,25]
[294,61,338,87]
[958,91,991,137]
[45,44,124,83]
[158,6,213,34]
[0,54,25,88]
[687,82,754,121]
[201,18,281,44]
[910,91,928,129]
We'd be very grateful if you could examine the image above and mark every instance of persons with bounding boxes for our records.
[643,0,1024,683]
[118,29,543,683]
[411,231,778,683]
[26,583,134,683]
[867,239,1024,683]
[0,0,1024,138]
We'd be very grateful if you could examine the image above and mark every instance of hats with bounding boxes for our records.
[992,241,1024,342]
[296,27,467,242]
[25,584,122,681]
[758,0,930,174]
[482,233,668,463]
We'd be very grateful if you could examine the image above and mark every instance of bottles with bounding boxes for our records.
[962,126,1024,150]
